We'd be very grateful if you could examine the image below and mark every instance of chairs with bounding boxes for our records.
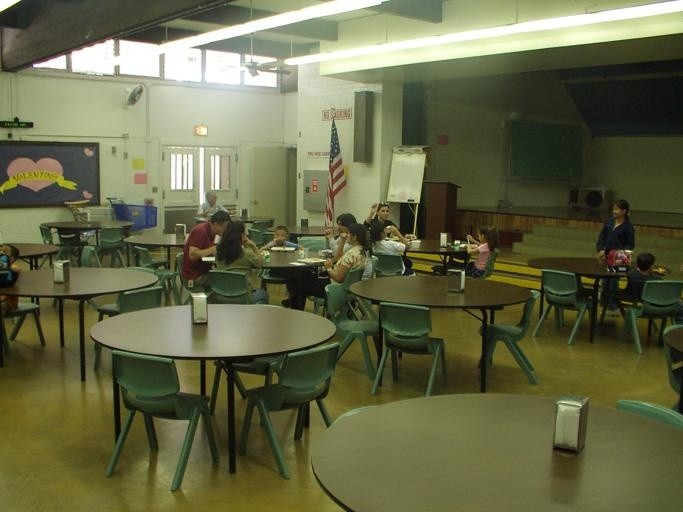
[207,351,286,429]
[368,299,448,397]
[117,285,164,314]
[531,268,597,346]
[0,300,49,355]
[204,268,249,304]
[131,243,170,268]
[237,339,341,479]
[618,278,683,356]
[469,247,500,278]
[102,348,219,493]
[79,244,97,266]
[660,323,682,411]
[475,289,542,386]
[613,397,682,430]
[295,233,328,253]
[369,248,406,278]
[36,223,54,244]
[323,282,381,383]
[95,225,125,266]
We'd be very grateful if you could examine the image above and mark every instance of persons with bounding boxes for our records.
[0,241,22,311]
[314,212,376,321]
[466,223,496,277]
[362,200,399,241]
[595,198,636,260]
[195,190,231,219]
[280,222,368,311]
[369,221,417,276]
[617,251,665,304]
[213,219,271,305]
[260,224,302,251]
[180,210,232,293]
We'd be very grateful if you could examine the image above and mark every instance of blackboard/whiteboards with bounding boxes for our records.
[507,119,585,180]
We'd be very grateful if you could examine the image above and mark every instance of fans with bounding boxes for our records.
[121,83,144,110]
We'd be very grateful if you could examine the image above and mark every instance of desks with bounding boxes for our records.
[39,218,138,232]
[402,238,472,270]
[0,241,61,268]
[309,391,683,512]
[264,224,341,237]
[120,233,223,247]
[0,266,161,381]
[202,245,333,269]
[87,302,338,476]
[524,255,672,341]
[347,271,536,394]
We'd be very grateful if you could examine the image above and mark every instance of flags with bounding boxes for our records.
[322,117,347,229]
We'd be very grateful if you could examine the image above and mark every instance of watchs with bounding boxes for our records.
[324,267,333,273]
